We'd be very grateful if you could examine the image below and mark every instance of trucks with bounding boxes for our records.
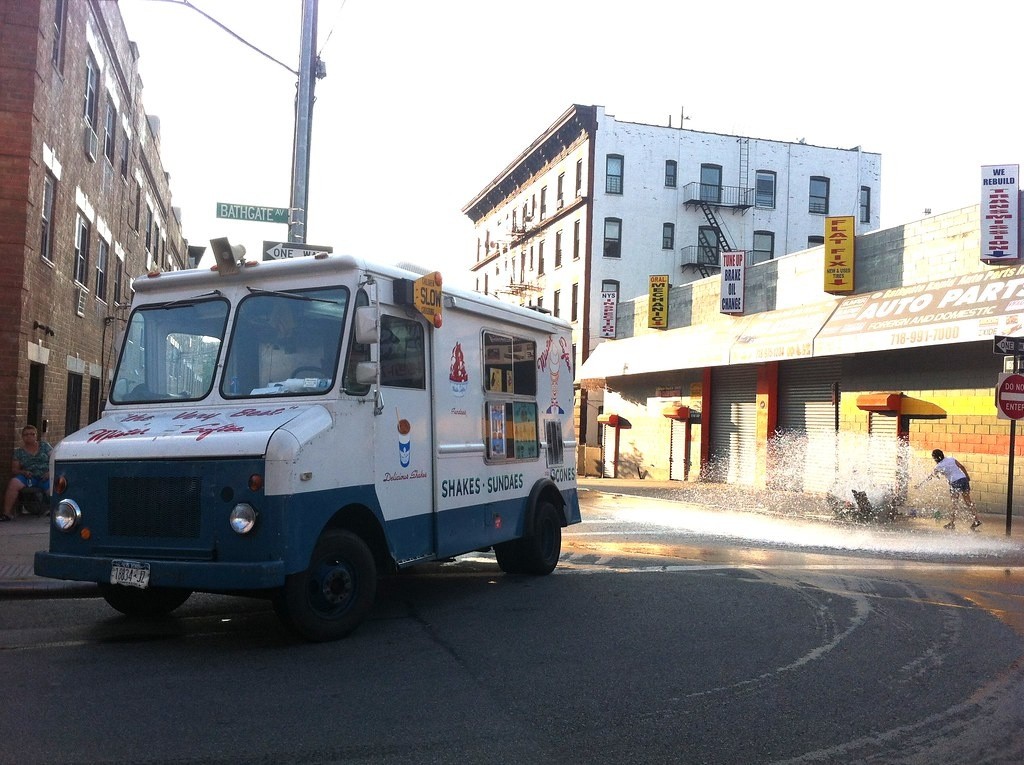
[36,238,582,639]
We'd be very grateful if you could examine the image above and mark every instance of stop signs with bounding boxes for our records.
[997,372,1024,420]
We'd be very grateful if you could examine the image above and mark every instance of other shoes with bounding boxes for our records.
[970,521,982,529]
[944,523,955,530]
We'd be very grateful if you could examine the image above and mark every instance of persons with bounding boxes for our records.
[914,448,984,531]
[0,424,54,522]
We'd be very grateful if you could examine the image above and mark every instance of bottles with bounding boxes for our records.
[230,376,242,396]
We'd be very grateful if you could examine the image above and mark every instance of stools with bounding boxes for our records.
[16,486,53,518]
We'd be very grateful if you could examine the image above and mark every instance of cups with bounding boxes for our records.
[397,419,411,467]
